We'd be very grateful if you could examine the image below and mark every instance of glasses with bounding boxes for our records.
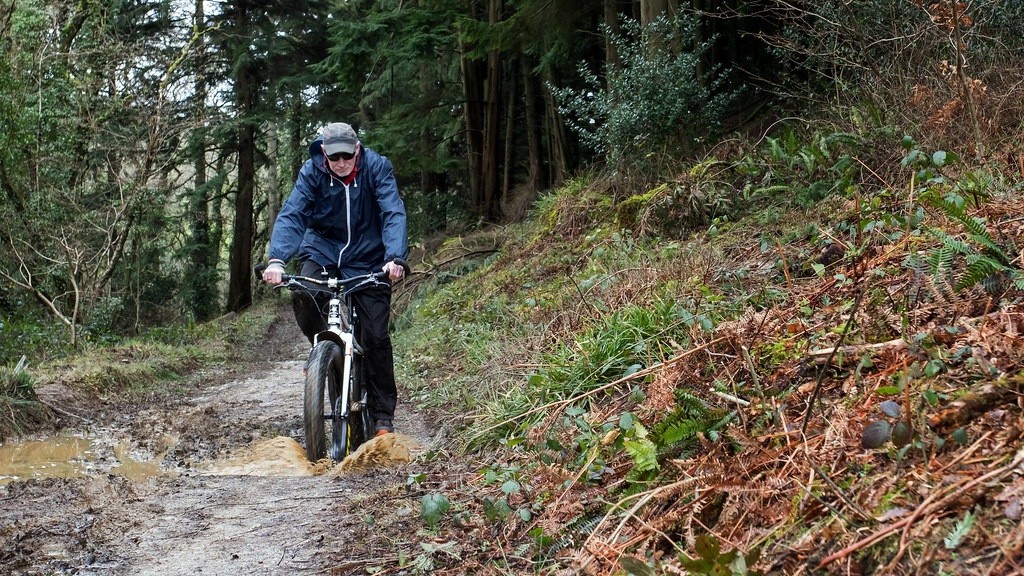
[324,150,356,161]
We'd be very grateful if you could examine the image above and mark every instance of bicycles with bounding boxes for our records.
[257,256,414,469]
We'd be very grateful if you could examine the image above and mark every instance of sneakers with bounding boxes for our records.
[372,419,394,438]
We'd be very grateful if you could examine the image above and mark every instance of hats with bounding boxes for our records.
[322,122,358,155]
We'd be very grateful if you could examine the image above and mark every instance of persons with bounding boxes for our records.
[262,121,411,443]
